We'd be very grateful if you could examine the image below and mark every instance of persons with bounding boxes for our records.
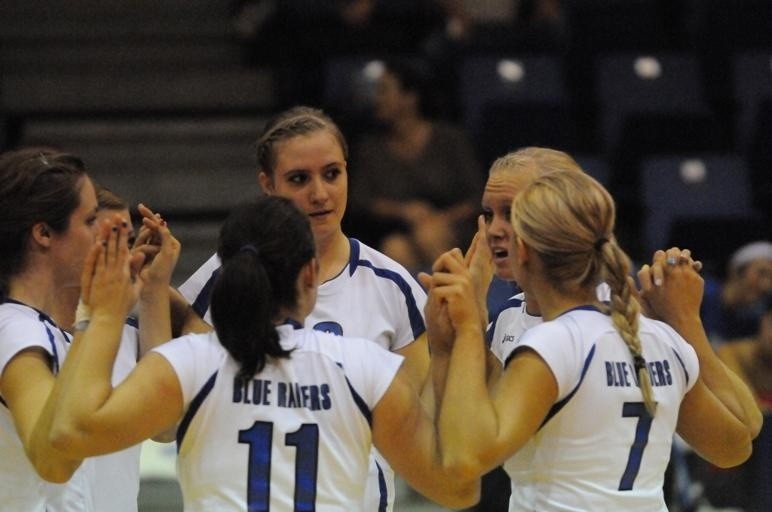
[0,108,763,512]
[235,0,607,275]
[697,241,772,512]
